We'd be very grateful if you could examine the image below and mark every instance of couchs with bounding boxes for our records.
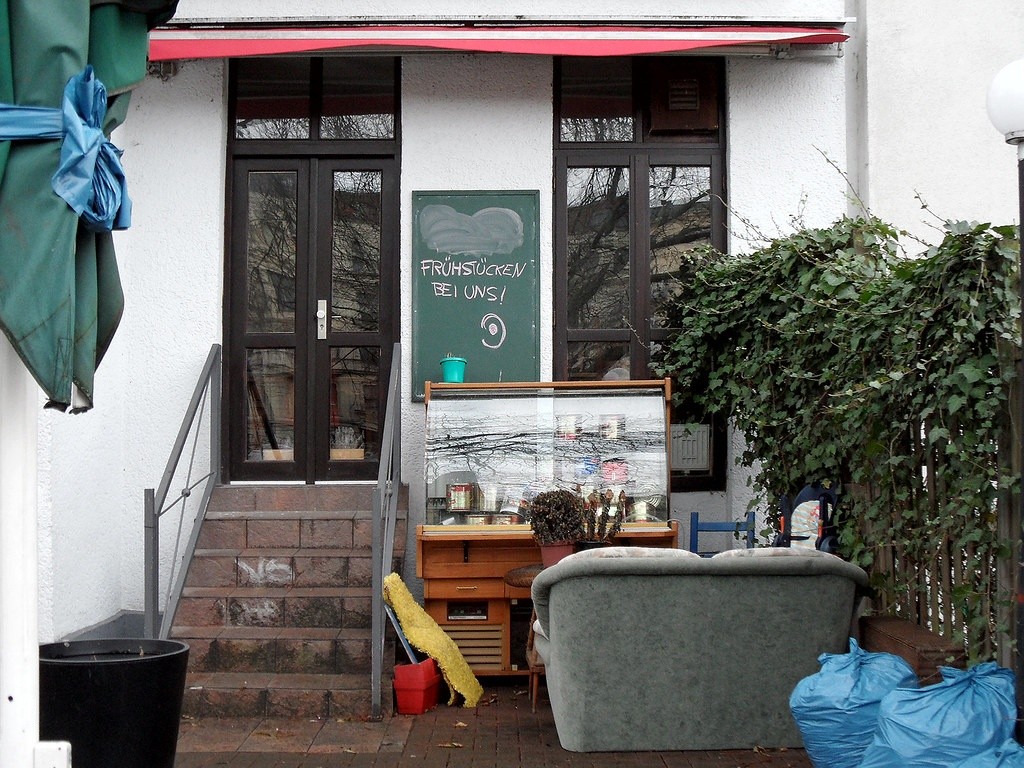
[531,547,870,753]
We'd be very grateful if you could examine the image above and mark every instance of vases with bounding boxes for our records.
[440,357,467,383]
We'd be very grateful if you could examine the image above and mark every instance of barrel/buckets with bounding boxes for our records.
[439,357,468,383]
[445,483,474,512]
[554,413,582,440]
[38,637,191,768]
[463,514,521,526]
[498,485,531,517]
[629,501,654,522]
[574,454,601,478]
[598,413,626,441]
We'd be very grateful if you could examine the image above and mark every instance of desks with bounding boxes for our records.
[504,564,545,714]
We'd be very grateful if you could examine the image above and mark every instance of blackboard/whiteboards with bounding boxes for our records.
[411,189,542,402]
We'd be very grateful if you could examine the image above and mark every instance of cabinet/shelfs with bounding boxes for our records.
[417,520,678,677]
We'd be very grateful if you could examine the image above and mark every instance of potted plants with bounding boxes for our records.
[528,488,626,568]
